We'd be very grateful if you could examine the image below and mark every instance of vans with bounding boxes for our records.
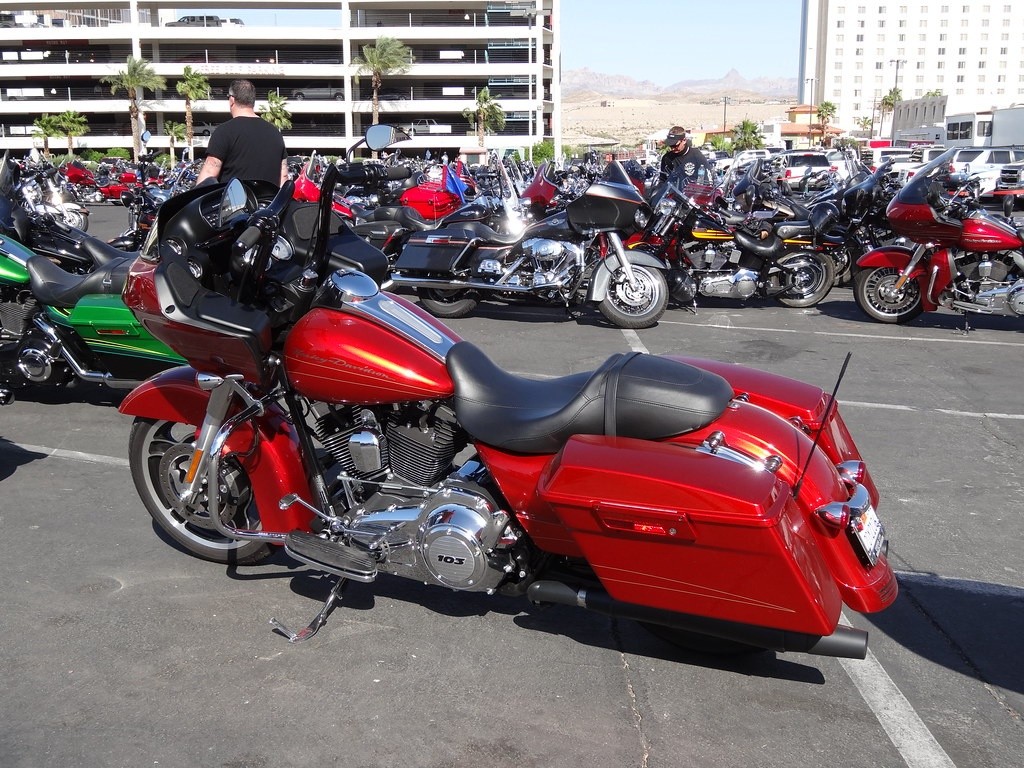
[291,81,345,100]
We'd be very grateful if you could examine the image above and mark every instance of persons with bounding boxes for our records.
[660,126,711,191]
[196,80,289,189]
[442,152,448,164]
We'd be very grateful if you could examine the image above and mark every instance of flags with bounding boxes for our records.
[456,161,476,188]
[442,164,468,204]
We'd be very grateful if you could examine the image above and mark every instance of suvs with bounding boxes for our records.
[165,16,221,27]
[861,147,1024,196]
[218,18,245,27]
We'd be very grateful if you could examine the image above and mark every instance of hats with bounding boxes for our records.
[664,134,686,146]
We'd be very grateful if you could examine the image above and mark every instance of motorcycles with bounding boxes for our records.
[0,146,200,279]
[853,147,1024,334]
[288,146,661,289]
[391,156,668,330]
[0,233,188,404]
[642,155,900,317]
[118,126,900,663]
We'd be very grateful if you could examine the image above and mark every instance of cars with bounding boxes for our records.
[703,147,859,191]
[366,88,411,101]
[398,119,440,136]
[192,121,218,136]
[1,88,28,101]
[997,158,1024,190]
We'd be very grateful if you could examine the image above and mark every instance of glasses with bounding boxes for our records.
[227,94,238,101]
